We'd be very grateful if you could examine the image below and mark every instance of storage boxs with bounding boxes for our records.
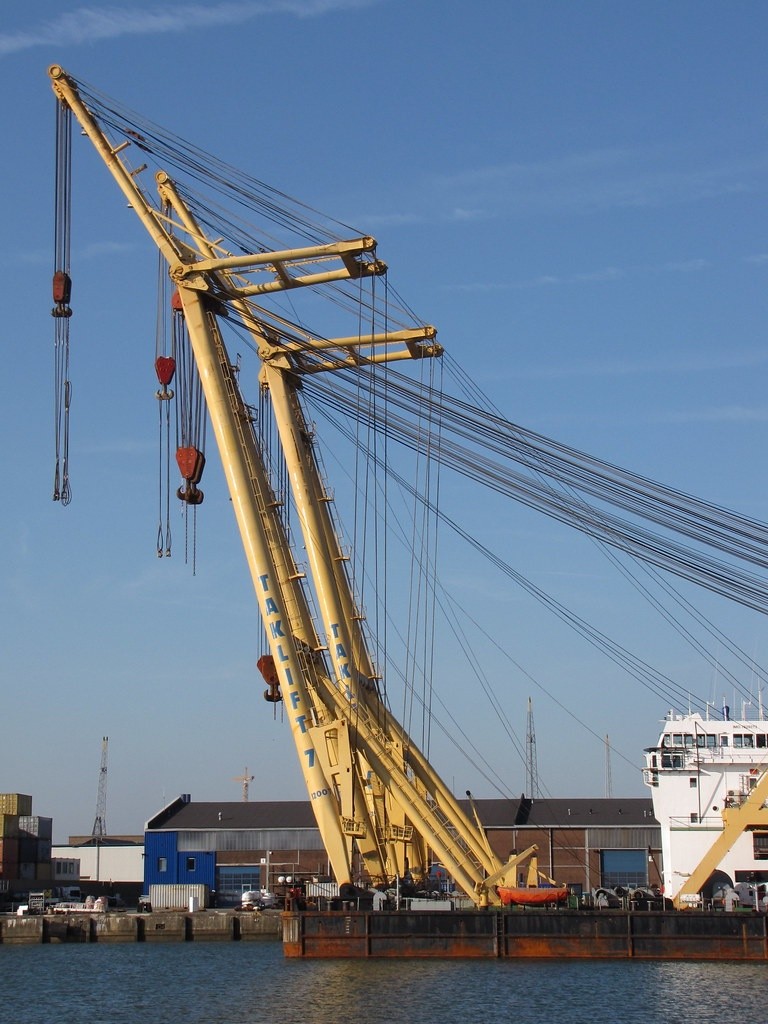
[0,793,54,901]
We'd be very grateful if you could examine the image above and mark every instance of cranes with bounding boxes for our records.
[93,736,110,837]
[231,766,254,801]
[36,63,545,907]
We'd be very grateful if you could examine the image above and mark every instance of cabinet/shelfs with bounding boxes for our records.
[269,862,321,901]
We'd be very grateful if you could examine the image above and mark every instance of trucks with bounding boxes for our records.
[59,886,82,902]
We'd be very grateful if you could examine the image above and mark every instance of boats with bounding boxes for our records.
[498,885,568,909]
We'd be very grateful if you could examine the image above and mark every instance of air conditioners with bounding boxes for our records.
[726,789,741,805]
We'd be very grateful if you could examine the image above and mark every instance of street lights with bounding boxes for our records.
[266,849,274,897]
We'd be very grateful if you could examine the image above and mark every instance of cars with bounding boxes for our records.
[235,904,264,912]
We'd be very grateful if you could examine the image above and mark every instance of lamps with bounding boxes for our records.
[588,809,592,814]
[618,809,621,814]
